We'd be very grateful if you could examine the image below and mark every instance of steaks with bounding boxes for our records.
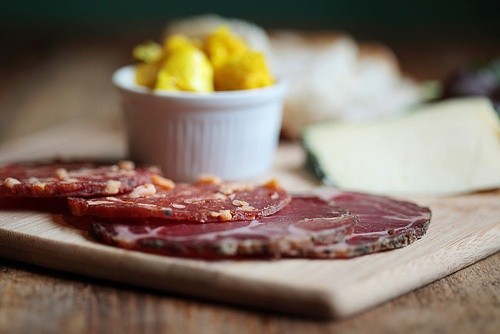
[0,155,431,259]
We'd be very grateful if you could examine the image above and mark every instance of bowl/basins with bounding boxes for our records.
[110,57,290,195]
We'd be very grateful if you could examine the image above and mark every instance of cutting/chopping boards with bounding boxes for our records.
[0,129,499,319]
